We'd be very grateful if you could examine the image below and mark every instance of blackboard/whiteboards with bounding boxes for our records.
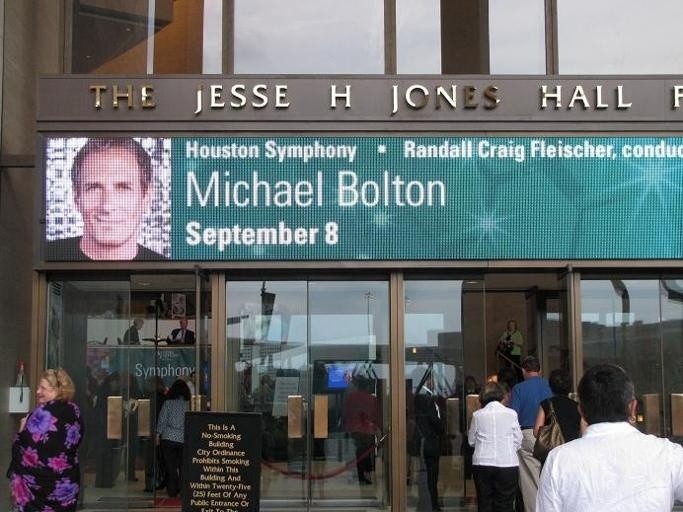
[182,412,262,512]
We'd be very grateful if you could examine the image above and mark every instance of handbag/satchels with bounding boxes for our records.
[534,411,565,463]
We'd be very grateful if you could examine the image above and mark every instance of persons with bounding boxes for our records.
[509,354,557,511]
[533,368,589,476]
[496,320,526,381]
[467,381,523,511]
[343,376,383,486]
[462,376,480,395]
[247,374,287,462]
[167,318,194,347]
[5,367,88,512]
[44,137,171,260]
[411,364,448,512]
[89,369,210,498]
[120,315,144,346]
[535,363,683,512]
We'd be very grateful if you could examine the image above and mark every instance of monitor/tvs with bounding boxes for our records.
[312,359,381,397]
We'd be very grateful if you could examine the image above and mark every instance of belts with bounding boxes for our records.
[522,425,533,430]
